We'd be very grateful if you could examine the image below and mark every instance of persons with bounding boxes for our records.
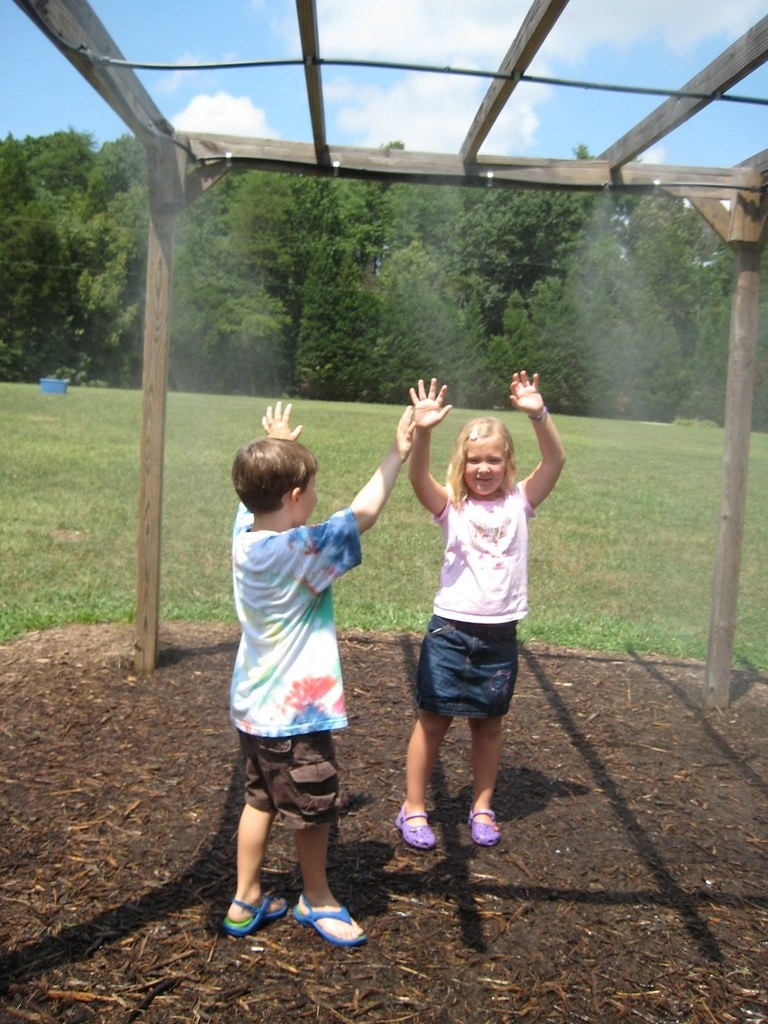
[396,369,567,848]
[220,401,416,946]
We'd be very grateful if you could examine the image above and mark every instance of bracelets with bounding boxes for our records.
[528,406,548,419]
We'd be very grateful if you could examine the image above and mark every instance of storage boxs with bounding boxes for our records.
[40,378,68,394]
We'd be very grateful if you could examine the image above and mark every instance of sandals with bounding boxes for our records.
[468,803,500,846]
[222,894,288,936]
[294,890,367,946]
[396,799,436,849]
